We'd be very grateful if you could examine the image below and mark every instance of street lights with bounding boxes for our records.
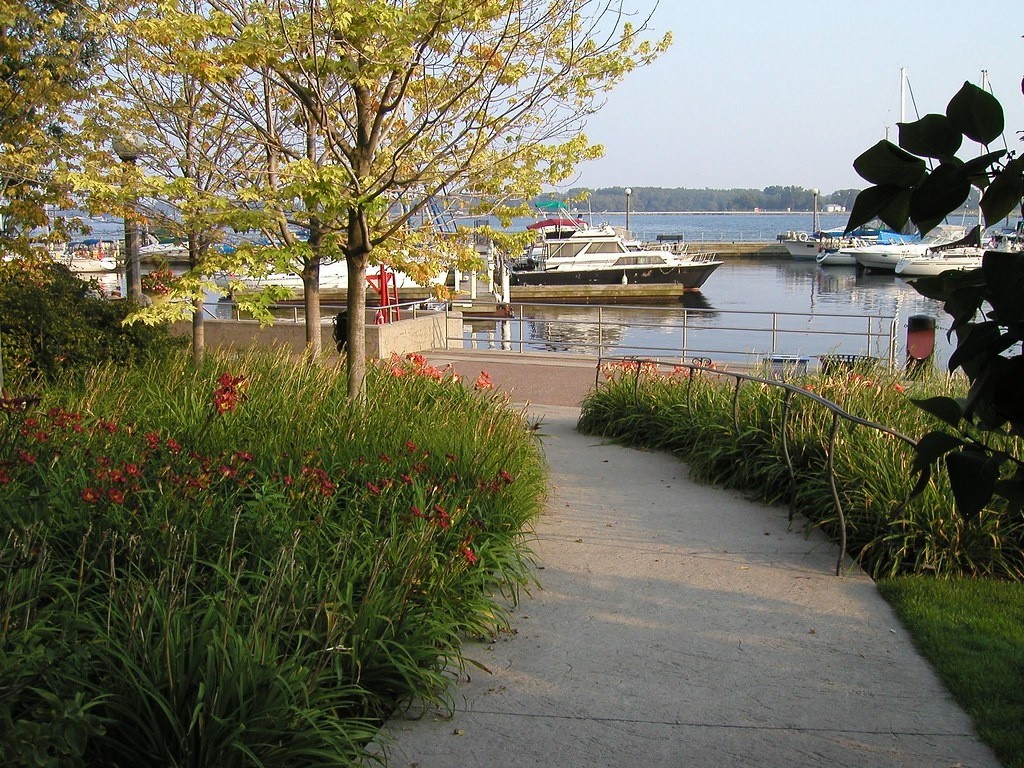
[812,188,819,232]
[625,187,632,230]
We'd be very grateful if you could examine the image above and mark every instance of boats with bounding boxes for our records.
[781,67,1021,278]
[5,197,728,293]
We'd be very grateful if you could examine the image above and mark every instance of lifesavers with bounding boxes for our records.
[798,233,808,242]
